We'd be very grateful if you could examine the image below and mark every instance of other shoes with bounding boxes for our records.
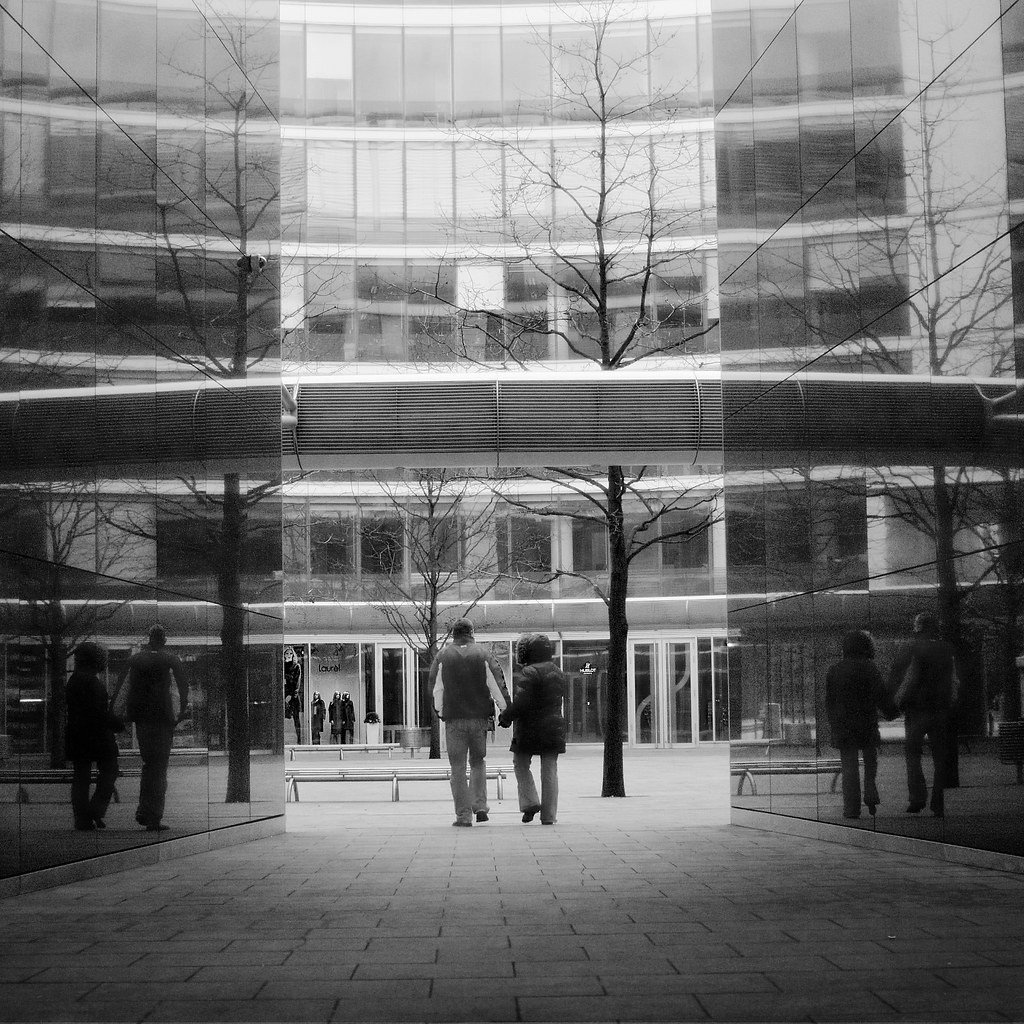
[543,821,553,825]
[906,798,926,813]
[930,803,945,821]
[89,813,107,829]
[146,822,170,831]
[136,815,148,826]
[476,811,489,822]
[73,824,88,832]
[521,805,540,823]
[843,809,860,819]
[452,822,471,827]
[863,796,877,816]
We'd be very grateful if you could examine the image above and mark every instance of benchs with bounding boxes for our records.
[284,764,515,802]
[284,743,401,761]
[730,757,865,796]
[0,768,143,804]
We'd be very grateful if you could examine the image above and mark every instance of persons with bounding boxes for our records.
[311,691,355,744]
[65,625,189,830]
[429,618,511,826]
[284,646,302,745]
[500,633,567,825]
[826,612,959,818]
